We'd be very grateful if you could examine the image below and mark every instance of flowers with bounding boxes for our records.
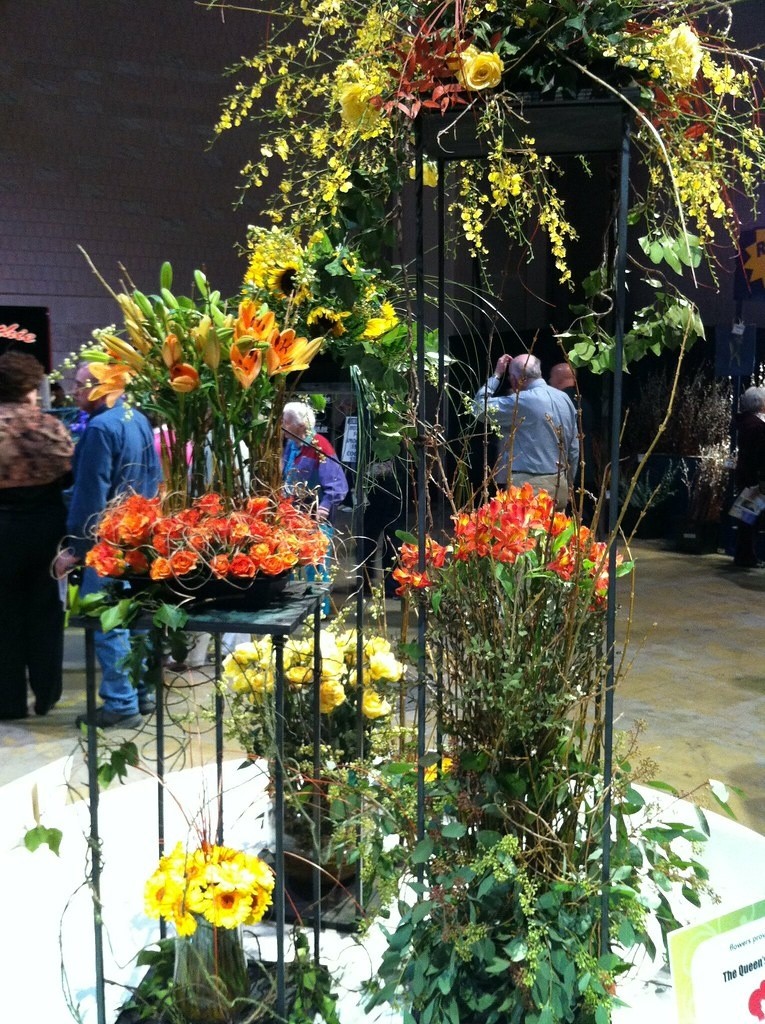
[49,244,326,510]
[222,628,408,771]
[394,482,634,832]
[239,223,459,465]
[142,842,275,937]
[63,488,330,635]
[203,0,765,376]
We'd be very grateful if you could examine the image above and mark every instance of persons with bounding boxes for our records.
[470,354,580,511]
[0,352,76,721]
[152,422,250,668]
[55,362,164,729]
[731,386,765,569]
[548,363,598,528]
[281,402,349,618]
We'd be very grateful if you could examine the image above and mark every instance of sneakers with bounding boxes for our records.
[75,696,156,731]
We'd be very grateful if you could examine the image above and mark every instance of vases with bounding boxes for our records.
[172,917,248,1024]
[109,574,290,607]
[268,747,372,886]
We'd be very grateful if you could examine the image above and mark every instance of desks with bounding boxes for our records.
[1,757,765,1024]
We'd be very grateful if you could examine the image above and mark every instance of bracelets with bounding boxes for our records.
[492,374,501,382]
